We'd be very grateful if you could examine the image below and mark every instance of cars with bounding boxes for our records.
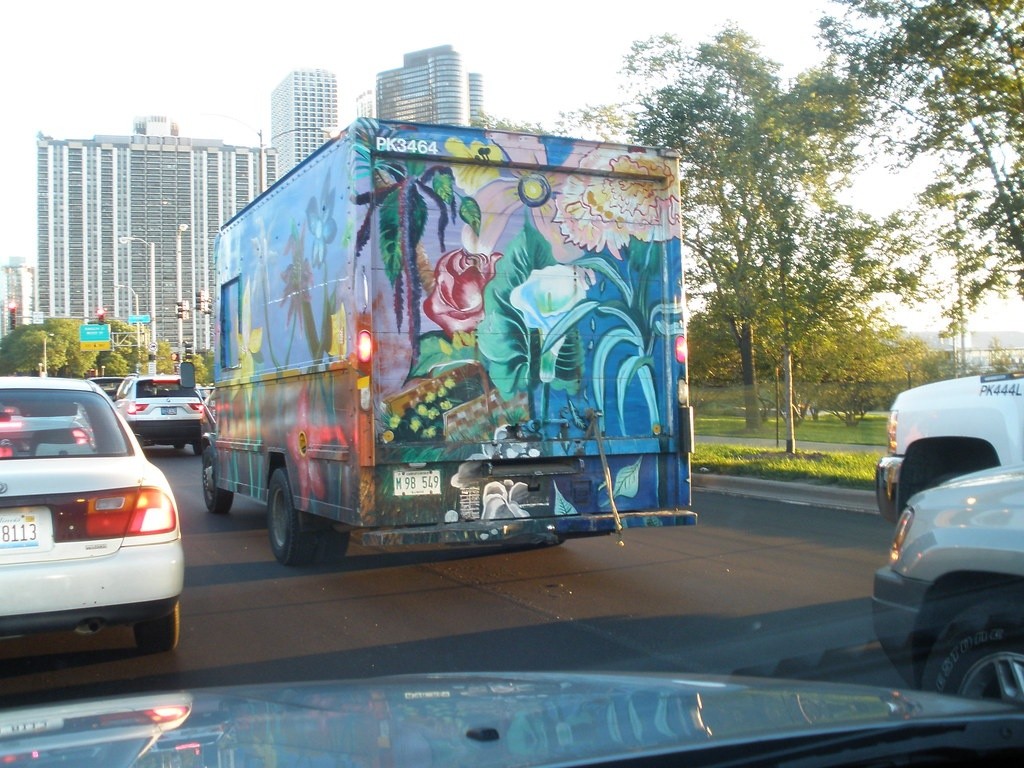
[866,464,1024,710]
[0,374,185,654]
[0,401,96,457]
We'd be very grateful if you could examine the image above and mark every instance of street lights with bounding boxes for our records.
[42,333,54,378]
[172,223,189,368]
[255,125,340,195]
[119,237,158,375]
[113,285,142,363]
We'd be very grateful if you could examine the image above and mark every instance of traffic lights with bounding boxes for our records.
[183,341,194,361]
[8,302,18,331]
[193,290,204,310]
[176,300,190,320]
[205,296,214,314]
[171,351,179,361]
[97,307,105,325]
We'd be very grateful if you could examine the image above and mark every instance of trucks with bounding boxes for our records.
[175,114,699,568]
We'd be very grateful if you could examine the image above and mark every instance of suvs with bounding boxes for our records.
[873,369,1024,525]
[87,375,214,456]
